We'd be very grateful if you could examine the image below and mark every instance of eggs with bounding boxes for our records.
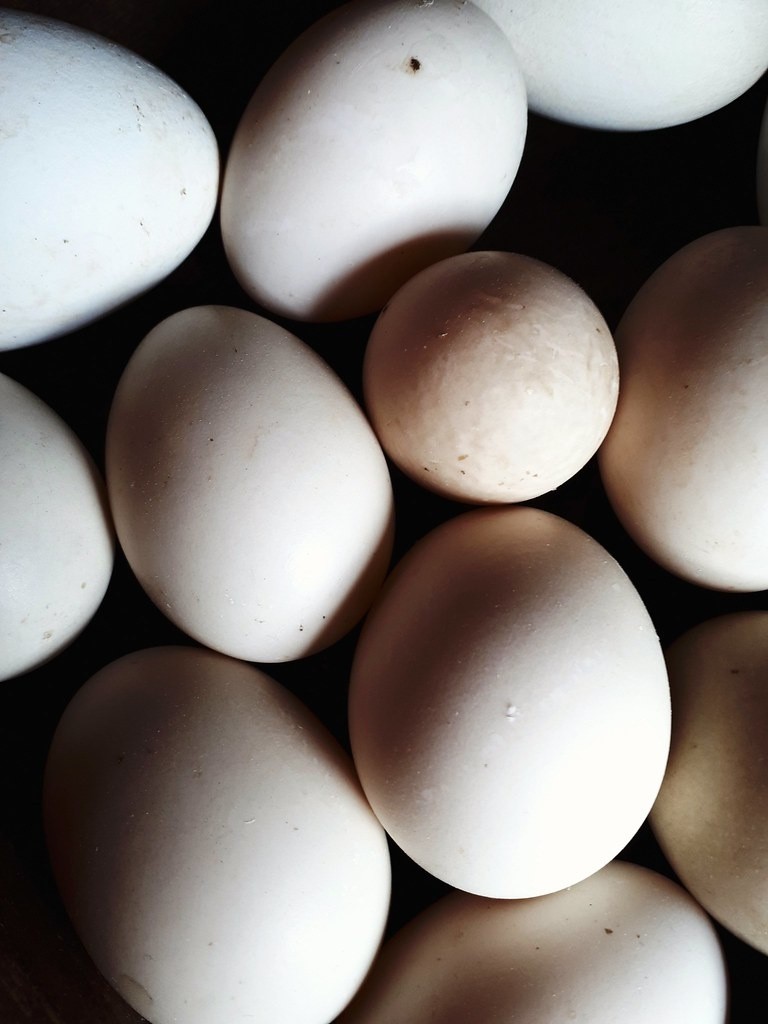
[0,0,768,1024]
